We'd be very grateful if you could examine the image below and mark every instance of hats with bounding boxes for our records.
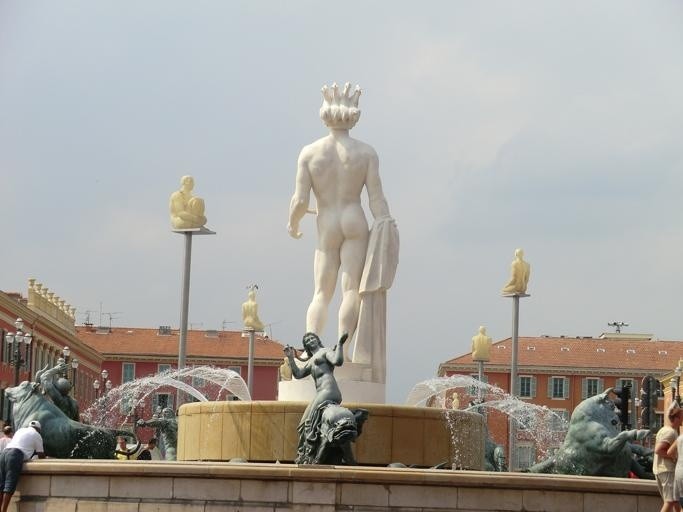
[29,421,42,429]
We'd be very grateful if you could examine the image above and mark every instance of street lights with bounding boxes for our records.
[5,315,34,387]
[94,367,113,399]
[54,344,79,399]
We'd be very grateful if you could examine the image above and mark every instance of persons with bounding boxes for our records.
[168,175,208,231]
[285,119,397,364]
[470,324,492,360]
[35,359,81,422]
[135,406,176,459]
[485,446,508,471]
[242,290,266,331]
[499,248,531,293]
[0,420,47,512]
[113,436,136,460]
[134,437,157,460]
[666,432,682,503]
[282,331,349,456]
[0,425,13,455]
[652,399,682,512]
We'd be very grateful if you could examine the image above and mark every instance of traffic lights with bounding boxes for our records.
[609,385,629,425]
